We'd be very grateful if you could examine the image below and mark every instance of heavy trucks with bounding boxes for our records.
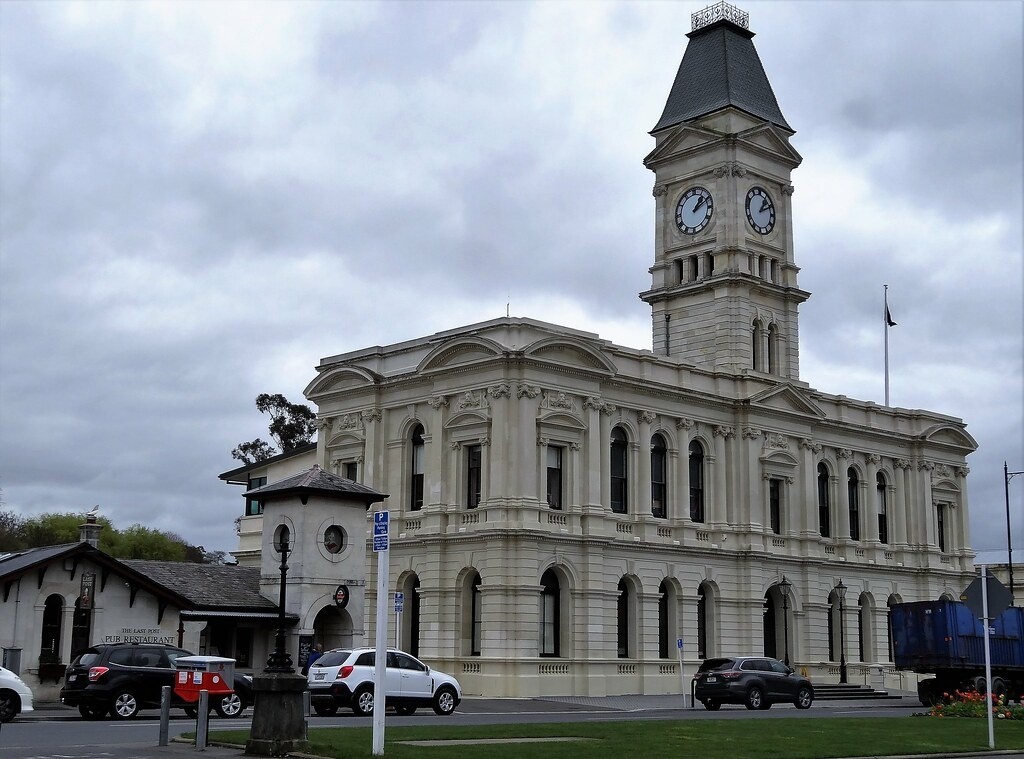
[887,601,1024,708]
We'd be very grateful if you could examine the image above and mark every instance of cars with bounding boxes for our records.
[0,666,35,723]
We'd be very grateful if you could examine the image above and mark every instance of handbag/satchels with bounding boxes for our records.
[301,663,308,676]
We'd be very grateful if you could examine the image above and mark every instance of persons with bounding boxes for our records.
[302,643,324,674]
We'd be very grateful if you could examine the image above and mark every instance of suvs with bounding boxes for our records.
[60,639,256,722]
[307,645,464,717]
[694,656,814,711]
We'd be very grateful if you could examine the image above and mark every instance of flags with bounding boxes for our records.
[887,307,898,327]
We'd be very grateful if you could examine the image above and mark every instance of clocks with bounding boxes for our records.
[675,187,714,235]
[745,186,776,235]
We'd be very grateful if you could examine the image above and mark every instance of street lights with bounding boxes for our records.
[1003,460,1024,605]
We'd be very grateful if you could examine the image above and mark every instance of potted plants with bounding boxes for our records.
[38,649,67,677]
